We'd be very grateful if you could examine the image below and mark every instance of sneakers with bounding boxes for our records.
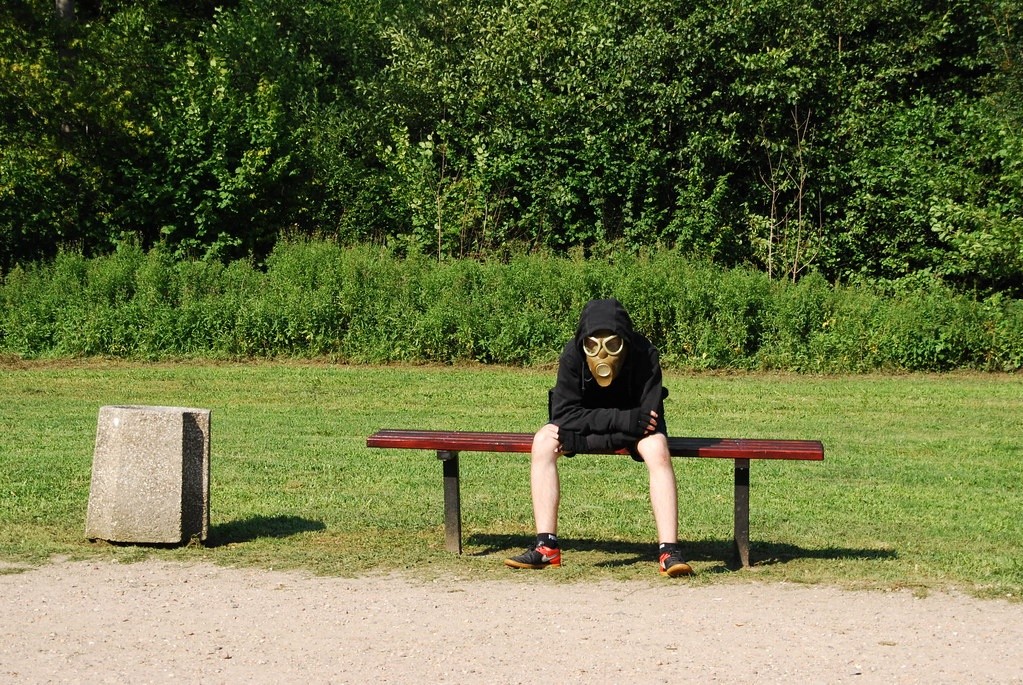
[658,543,693,576]
[504,541,561,568]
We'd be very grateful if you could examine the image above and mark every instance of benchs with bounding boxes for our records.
[365,427,824,571]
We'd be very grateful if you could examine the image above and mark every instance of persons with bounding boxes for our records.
[505,297,692,578]
